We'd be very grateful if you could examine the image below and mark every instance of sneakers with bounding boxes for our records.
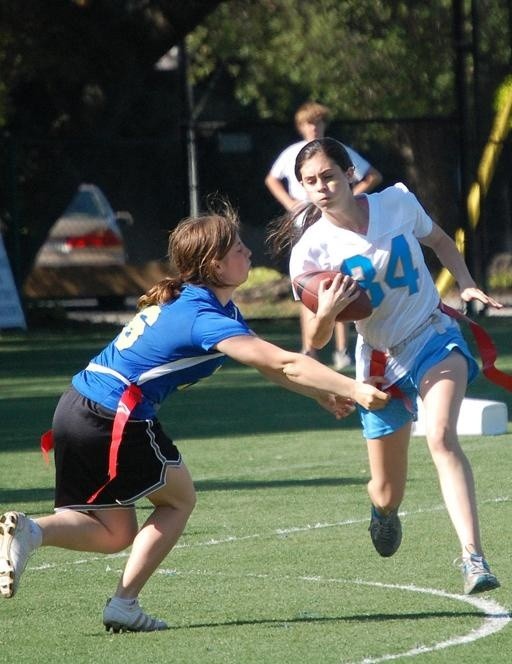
[0,511,32,598]
[102,598,168,633]
[367,503,403,557]
[462,554,501,595]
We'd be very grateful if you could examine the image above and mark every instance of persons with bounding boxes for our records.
[288,139,503,594]
[264,102,383,370]
[0,201,392,631]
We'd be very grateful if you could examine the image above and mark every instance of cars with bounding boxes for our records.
[21,182,134,310]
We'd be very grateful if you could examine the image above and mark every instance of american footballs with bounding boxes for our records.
[293,270,372,321]
